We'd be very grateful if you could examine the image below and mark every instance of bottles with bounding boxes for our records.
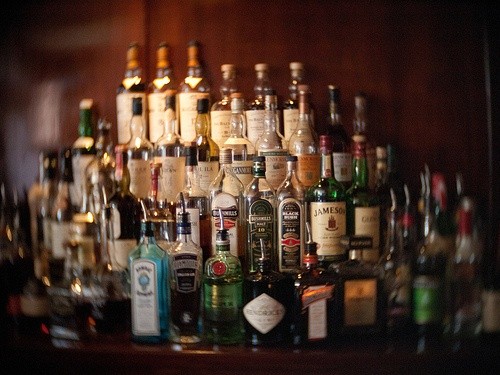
[0,40,499,354]
